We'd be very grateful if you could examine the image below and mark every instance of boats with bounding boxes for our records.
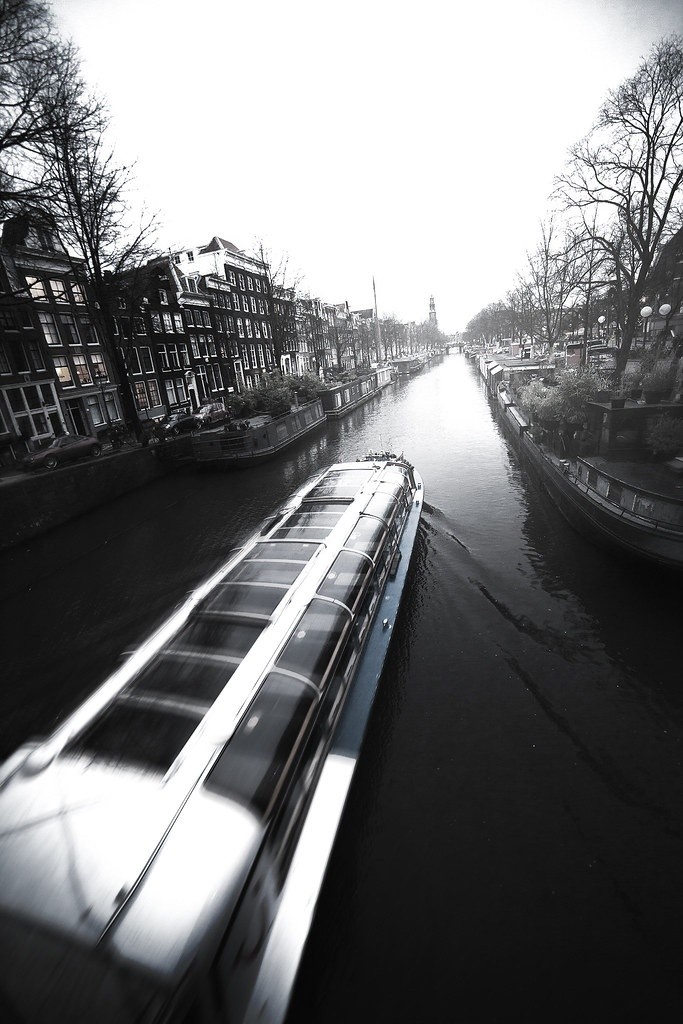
[0,450,424,1024]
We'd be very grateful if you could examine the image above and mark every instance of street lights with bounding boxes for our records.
[639,305,652,350]
[659,302,671,331]
[598,314,606,339]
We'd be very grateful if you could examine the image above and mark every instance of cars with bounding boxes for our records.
[21,434,101,471]
[153,403,227,442]
